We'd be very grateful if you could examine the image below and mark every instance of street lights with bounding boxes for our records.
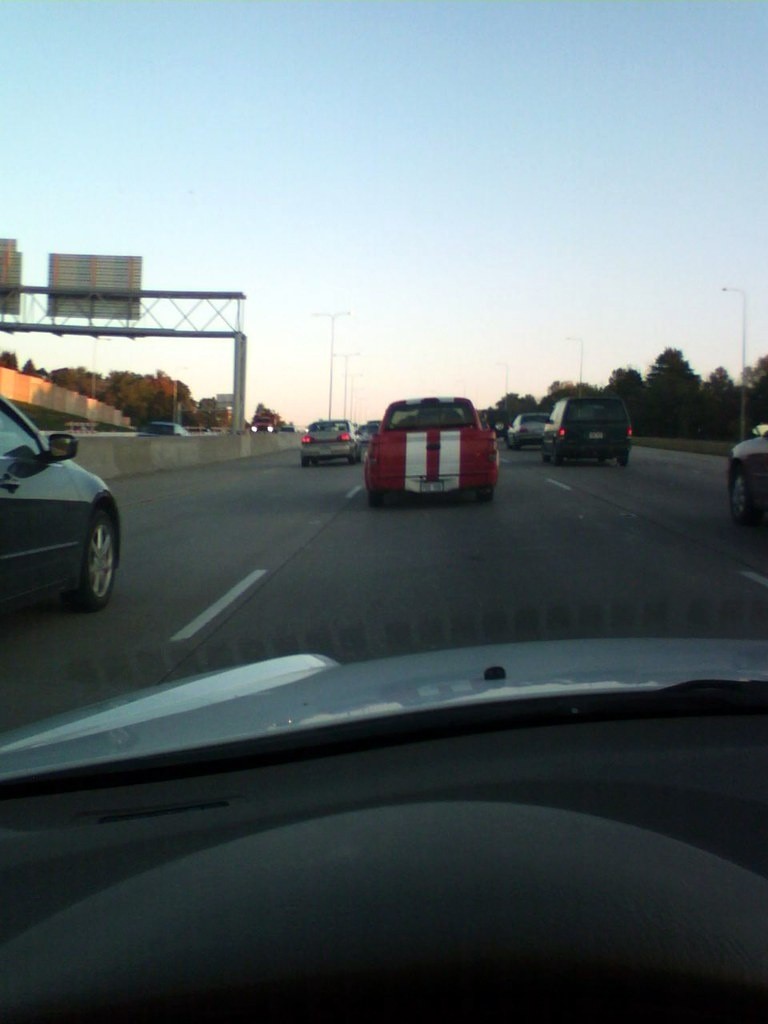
[312,311,351,421]
[722,286,746,441]
[333,350,360,420]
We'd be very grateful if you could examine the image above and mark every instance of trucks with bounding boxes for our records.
[250,412,279,432]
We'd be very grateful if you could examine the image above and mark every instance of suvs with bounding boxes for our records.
[485,409,509,440]
[542,395,634,466]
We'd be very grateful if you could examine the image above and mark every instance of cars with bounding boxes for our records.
[280,424,297,433]
[358,419,381,444]
[301,419,363,467]
[139,421,190,439]
[1,395,120,614]
[507,412,549,451]
[725,431,768,531]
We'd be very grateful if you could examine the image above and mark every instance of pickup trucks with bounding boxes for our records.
[364,396,499,504]
